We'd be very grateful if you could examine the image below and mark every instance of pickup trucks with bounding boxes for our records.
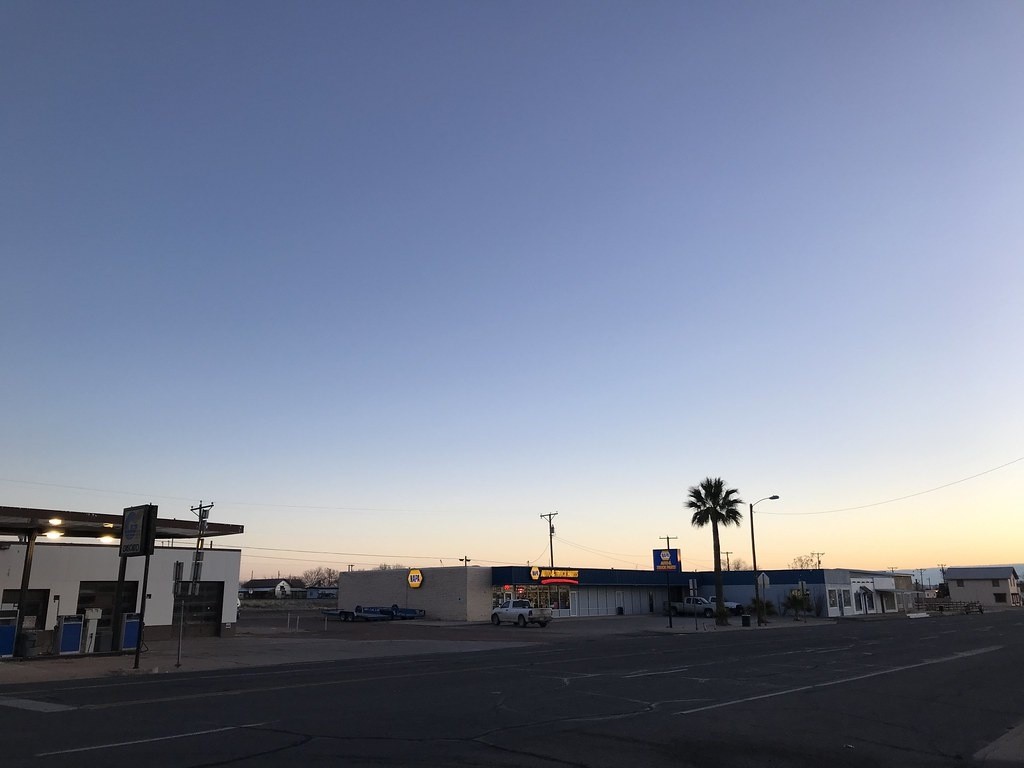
[663,597,726,618]
[491,599,553,627]
[704,597,743,615]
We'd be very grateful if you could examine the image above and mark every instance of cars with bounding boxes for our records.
[237,598,241,619]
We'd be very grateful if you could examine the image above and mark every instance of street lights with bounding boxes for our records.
[750,495,779,625]
